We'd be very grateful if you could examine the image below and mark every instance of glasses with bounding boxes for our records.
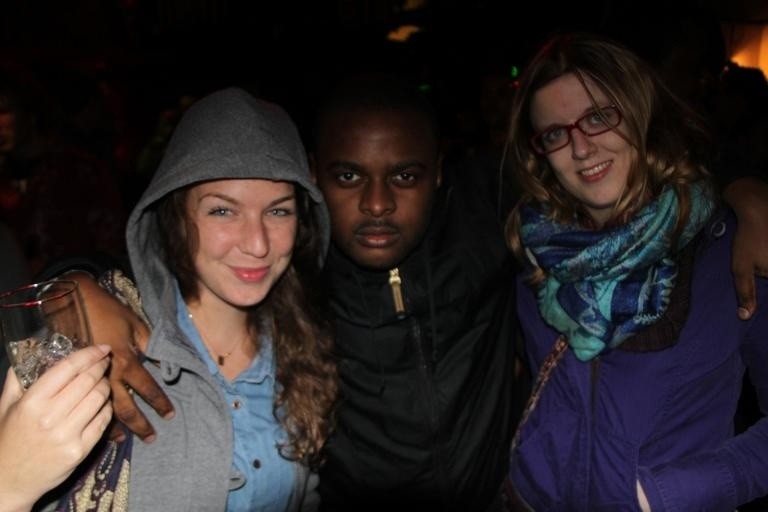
[531,105,621,156]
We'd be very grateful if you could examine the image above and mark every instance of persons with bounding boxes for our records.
[498,36,768,510]
[30,85,768,511]
[2,88,342,509]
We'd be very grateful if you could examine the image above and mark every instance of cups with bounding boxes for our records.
[0,281,96,396]
